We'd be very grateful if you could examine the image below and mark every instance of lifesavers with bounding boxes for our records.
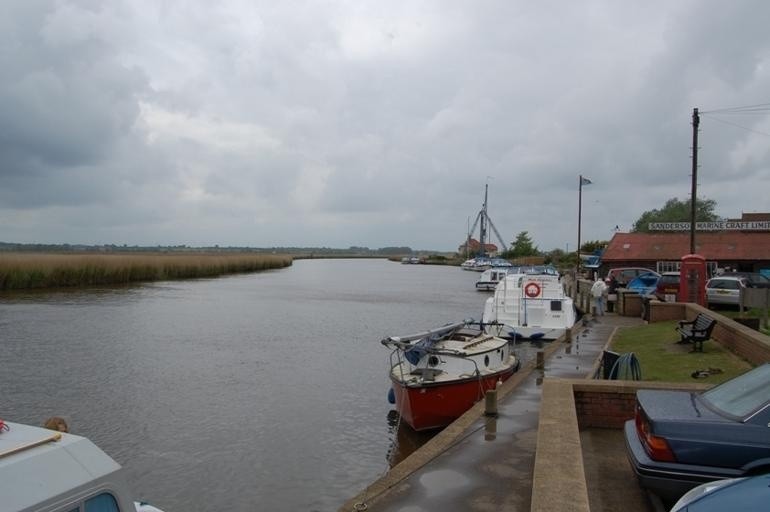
[525,283,540,297]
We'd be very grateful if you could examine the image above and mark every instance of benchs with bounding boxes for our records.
[676,312,717,352]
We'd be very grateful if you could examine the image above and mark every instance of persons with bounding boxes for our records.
[591,274,620,316]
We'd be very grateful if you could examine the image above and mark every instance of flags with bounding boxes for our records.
[581,176,593,185]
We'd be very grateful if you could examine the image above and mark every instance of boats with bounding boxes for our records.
[462,257,577,340]
[401,256,421,264]
[0,418,164,512]
[381,319,520,432]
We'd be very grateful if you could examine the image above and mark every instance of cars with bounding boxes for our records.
[705,271,770,311]
[605,266,679,302]
[622,358,770,511]
